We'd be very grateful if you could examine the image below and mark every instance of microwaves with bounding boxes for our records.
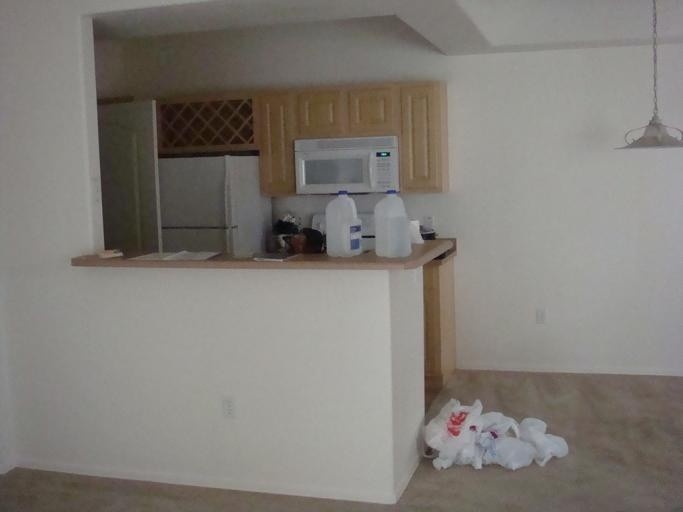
[293,136,401,195]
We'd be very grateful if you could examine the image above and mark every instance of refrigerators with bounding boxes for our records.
[157,153,273,259]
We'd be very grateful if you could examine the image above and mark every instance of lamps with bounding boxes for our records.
[621,0,682,147]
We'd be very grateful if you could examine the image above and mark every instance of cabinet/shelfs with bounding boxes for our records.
[155,81,450,197]
[423,251,454,390]
[69,241,455,505]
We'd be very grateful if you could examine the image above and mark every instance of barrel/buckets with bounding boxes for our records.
[372,188,413,257]
[326,189,364,259]
